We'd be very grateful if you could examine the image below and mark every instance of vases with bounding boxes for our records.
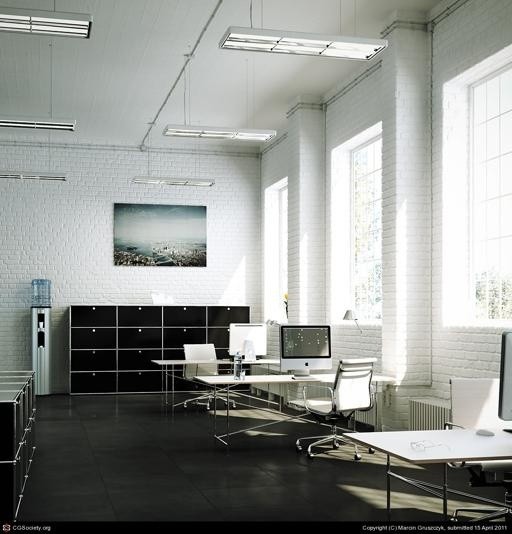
[286,304,288,319]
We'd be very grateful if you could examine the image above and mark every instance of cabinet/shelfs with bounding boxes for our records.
[1,370,37,522]
[68,303,251,395]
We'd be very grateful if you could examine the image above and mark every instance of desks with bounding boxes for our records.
[150,359,280,405]
[193,373,397,445]
[343,428,511,528]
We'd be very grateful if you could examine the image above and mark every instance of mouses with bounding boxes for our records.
[476,428,494,437]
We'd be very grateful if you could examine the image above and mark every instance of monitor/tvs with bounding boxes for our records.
[278,325,332,375]
[227,322,268,361]
[496,330,512,434]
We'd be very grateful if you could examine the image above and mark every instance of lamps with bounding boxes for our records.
[0,129,68,181]
[0,40,77,131]
[132,130,215,186]
[218,0,388,61]
[163,61,277,143]
[342,309,362,334]
[0,0,93,39]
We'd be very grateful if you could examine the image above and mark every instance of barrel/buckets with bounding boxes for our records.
[30,278,53,306]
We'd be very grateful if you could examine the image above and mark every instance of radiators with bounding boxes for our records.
[408,395,451,431]
[354,392,376,426]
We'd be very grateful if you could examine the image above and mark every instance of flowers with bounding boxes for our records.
[283,292,288,304]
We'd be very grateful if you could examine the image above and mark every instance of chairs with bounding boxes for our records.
[182,343,237,410]
[285,357,375,460]
[443,377,511,528]
